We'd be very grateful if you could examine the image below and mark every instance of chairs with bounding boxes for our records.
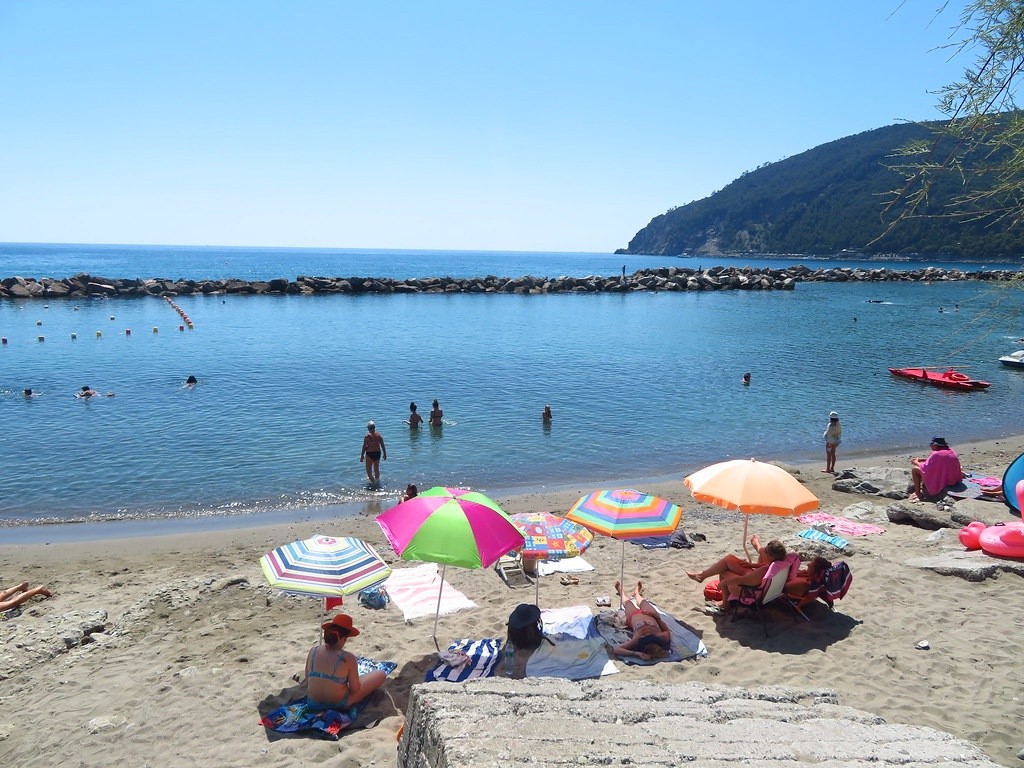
[495,555,535,588]
[730,551,853,639]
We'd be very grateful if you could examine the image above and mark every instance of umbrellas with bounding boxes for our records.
[374,483,530,633]
[684,457,821,545]
[505,511,595,610]
[564,484,683,612]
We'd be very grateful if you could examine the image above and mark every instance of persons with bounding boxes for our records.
[359,421,387,483]
[854,318,857,321]
[911,436,963,505]
[428,399,443,426]
[741,373,751,384]
[399,484,418,507]
[623,265,626,276]
[614,577,671,661]
[542,404,552,420]
[301,614,386,711]
[938,307,943,313]
[259,534,393,647]
[401,401,424,428]
[820,412,842,474]
[748,535,832,601]
[684,536,789,612]
[79,386,96,397]
[699,265,702,273]
[955,305,960,310]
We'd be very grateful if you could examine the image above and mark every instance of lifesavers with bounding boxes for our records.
[951,373,970,381]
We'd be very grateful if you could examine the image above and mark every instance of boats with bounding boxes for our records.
[999,351,1024,368]
[677,253,692,258]
[888,366,993,389]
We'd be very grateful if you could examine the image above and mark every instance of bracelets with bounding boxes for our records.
[632,651,634,656]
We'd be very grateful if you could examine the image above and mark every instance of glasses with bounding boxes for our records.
[929,444,933,446]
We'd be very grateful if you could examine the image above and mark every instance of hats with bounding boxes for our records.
[508,603,541,628]
[929,436,949,447]
[320,614,361,637]
[828,412,839,418]
